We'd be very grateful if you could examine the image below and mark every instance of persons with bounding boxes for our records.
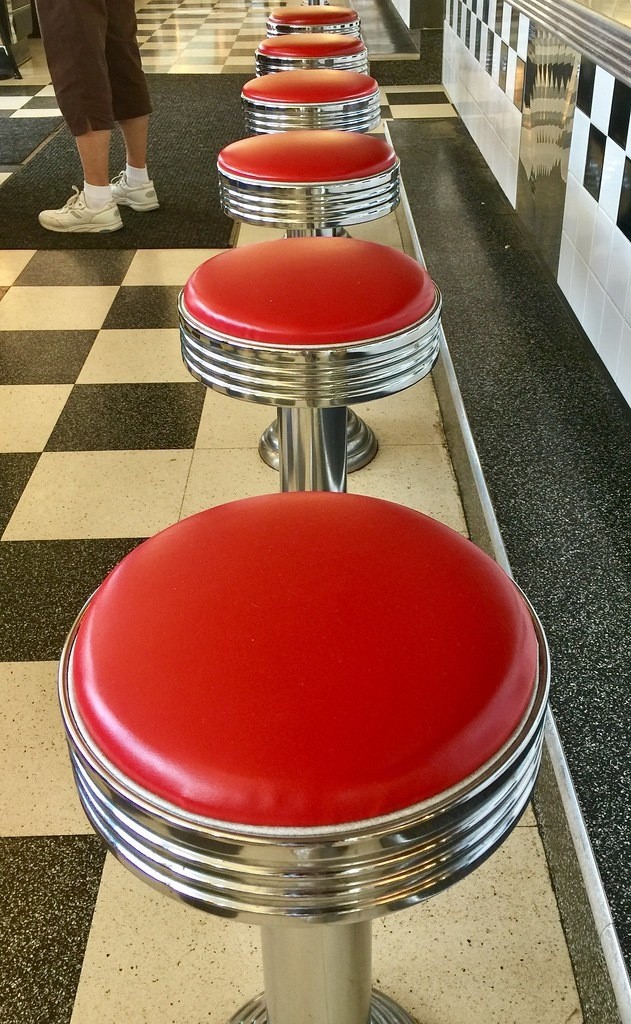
[36,0,160,235]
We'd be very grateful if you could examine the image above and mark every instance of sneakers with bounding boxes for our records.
[39,185,123,233]
[109,170,159,212]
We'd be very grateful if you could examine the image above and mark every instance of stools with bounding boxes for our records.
[267,5,362,40]
[214,129,400,238]
[177,235,447,495]
[61,490,554,1024]
[240,69,382,137]
[254,32,370,78]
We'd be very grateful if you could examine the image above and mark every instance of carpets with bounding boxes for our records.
[0,73,257,249]
[0,115,65,165]
